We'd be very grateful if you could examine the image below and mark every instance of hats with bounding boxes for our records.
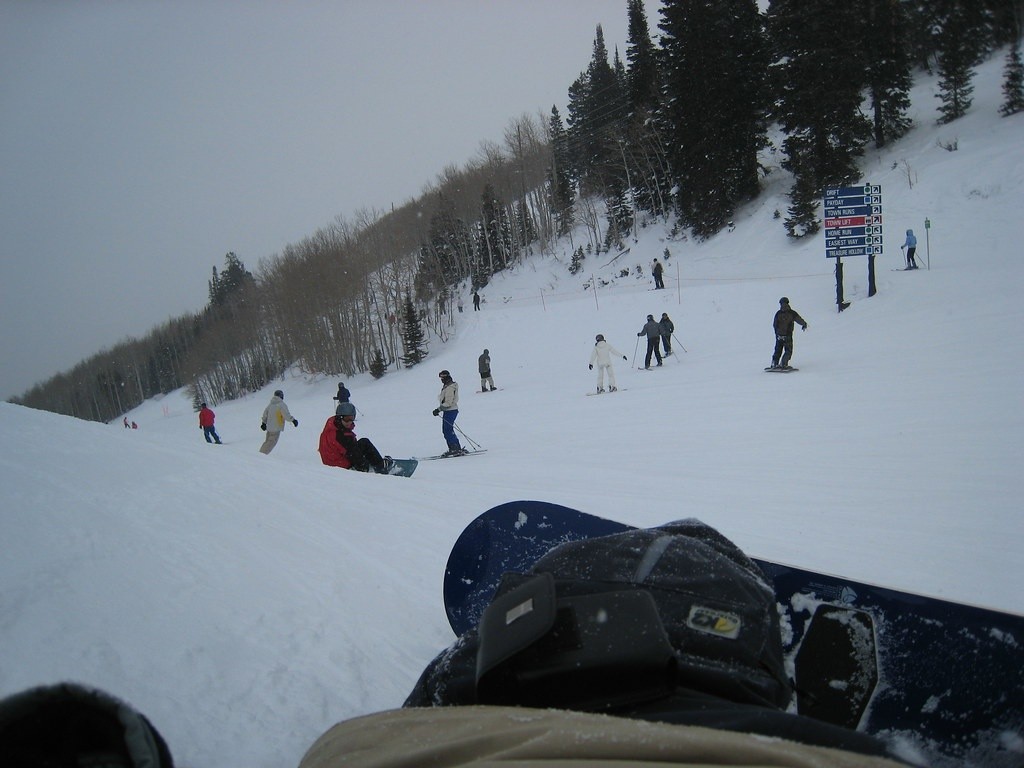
[647,315,654,320]
[338,382,344,387]
[476,519,794,715]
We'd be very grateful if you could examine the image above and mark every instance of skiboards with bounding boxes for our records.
[647,288,670,291]
[409,449,488,462]
[638,365,668,372]
[661,351,674,358]
[890,268,922,271]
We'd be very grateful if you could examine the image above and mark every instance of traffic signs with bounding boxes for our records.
[824,195,882,208]
[825,245,883,259]
[824,205,882,218]
[825,235,883,248]
[824,215,882,228]
[822,185,881,197]
[824,225,883,239]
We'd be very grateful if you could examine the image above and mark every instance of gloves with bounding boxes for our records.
[589,365,593,370]
[292,420,298,427]
[433,409,440,416]
[260,422,266,431]
[624,357,628,361]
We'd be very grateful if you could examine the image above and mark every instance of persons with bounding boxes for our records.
[389,313,395,324]
[901,229,918,270]
[651,258,664,290]
[589,335,628,394]
[319,402,394,472]
[637,314,662,369]
[433,370,459,456]
[660,314,674,356]
[0,516,915,767]
[771,296,809,368]
[457,297,463,312]
[124,417,130,428]
[259,389,298,455]
[333,382,350,404]
[478,349,497,392]
[472,292,481,311]
[199,403,221,444]
[132,421,137,428]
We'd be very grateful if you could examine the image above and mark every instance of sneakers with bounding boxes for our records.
[362,457,370,473]
[491,387,497,391]
[482,388,488,392]
[381,455,392,474]
[597,389,605,395]
[609,387,617,392]
[442,445,462,457]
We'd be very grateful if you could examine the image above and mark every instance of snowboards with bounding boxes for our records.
[349,459,419,477]
[765,367,800,374]
[441,497,1023,767]
[474,388,504,394]
[587,388,627,396]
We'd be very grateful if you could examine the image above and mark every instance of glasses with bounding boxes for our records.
[338,415,355,422]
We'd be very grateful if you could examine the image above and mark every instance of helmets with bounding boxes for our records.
[336,402,356,416]
[779,297,790,303]
[596,335,603,341]
[274,390,284,397]
